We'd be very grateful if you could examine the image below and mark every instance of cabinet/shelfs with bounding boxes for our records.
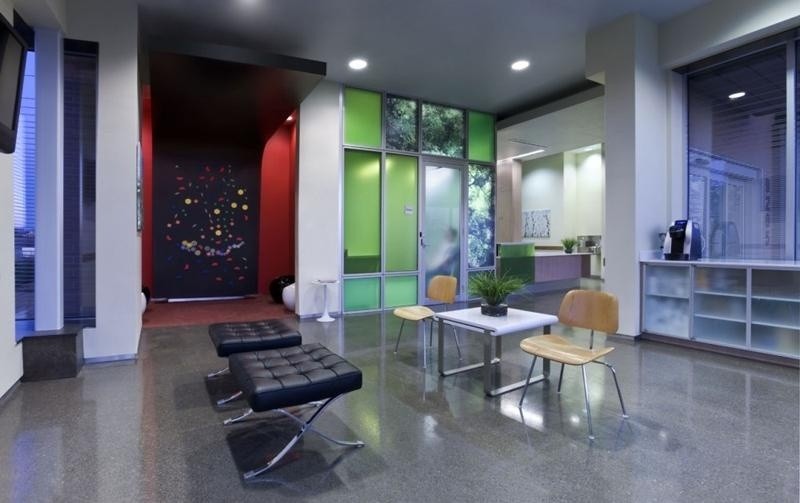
[639,249,800,370]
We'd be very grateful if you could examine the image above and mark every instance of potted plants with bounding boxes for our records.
[468,268,535,318]
[561,239,577,253]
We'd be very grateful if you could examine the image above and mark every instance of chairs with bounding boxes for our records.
[517,288,631,441]
[389,272,465,369]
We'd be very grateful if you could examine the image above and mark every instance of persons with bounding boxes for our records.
[423,226,460,297]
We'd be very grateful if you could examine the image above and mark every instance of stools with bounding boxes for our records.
[202,318,368,480]
